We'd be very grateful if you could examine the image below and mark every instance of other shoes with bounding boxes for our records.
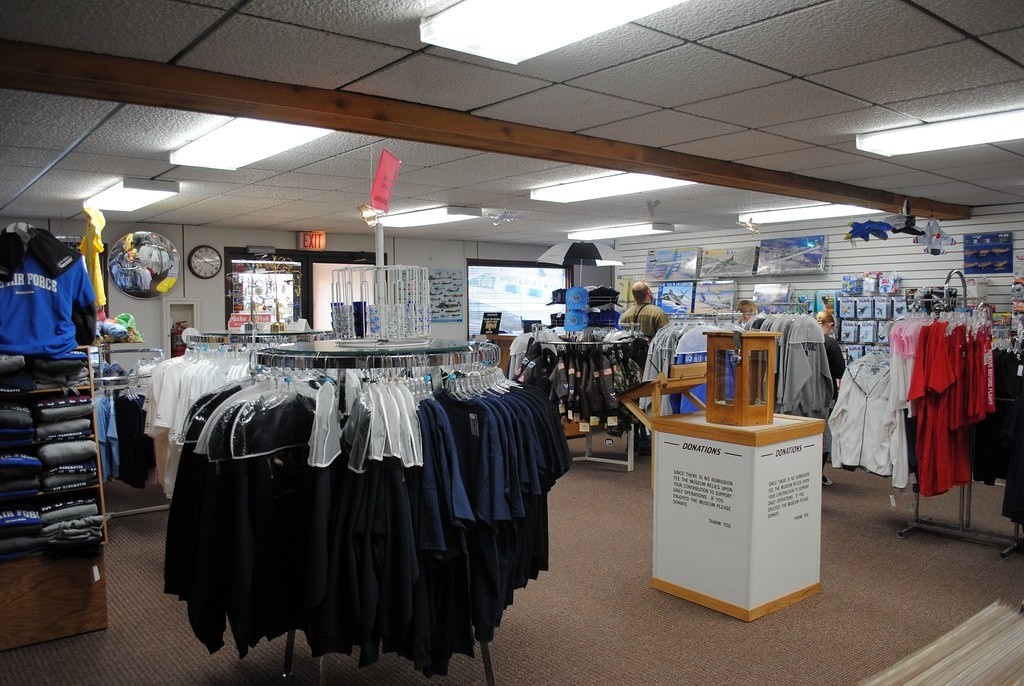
[822,474,832,485]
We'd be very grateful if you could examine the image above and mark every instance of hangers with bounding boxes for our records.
[529,328,640,360]
[668,300,811,327]
[92,358,161,404]
[116,240,170,273]
[855,347,890,374]
[7,222,34,234]
[184,333,520,418]
[905,300,1024,353]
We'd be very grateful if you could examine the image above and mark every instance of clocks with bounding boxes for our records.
[188,245,222,279]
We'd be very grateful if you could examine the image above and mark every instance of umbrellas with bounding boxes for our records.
[536,241,625,288]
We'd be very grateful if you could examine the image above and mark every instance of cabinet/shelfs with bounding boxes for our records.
[0,346,108,560]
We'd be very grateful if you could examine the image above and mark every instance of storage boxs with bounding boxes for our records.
[646,234,830,313]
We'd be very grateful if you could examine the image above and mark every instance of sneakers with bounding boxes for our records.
[626,443,651,455]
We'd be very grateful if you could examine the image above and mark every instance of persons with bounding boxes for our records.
[619,281,669,337]
[738,300,758,329]
[814,310,846,486]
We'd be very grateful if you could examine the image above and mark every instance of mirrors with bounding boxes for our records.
[108,231,183,300]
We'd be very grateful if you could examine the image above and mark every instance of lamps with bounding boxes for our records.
[82,180,179,213]
[569,224,676,242]
[530,172,697,204]
[373,207,483,229]
[418,0,687,65]
[170,117,336,172]
[739,204,884,225]
[856,108,1024,157]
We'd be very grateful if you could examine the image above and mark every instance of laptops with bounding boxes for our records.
[480,312,508,335]
